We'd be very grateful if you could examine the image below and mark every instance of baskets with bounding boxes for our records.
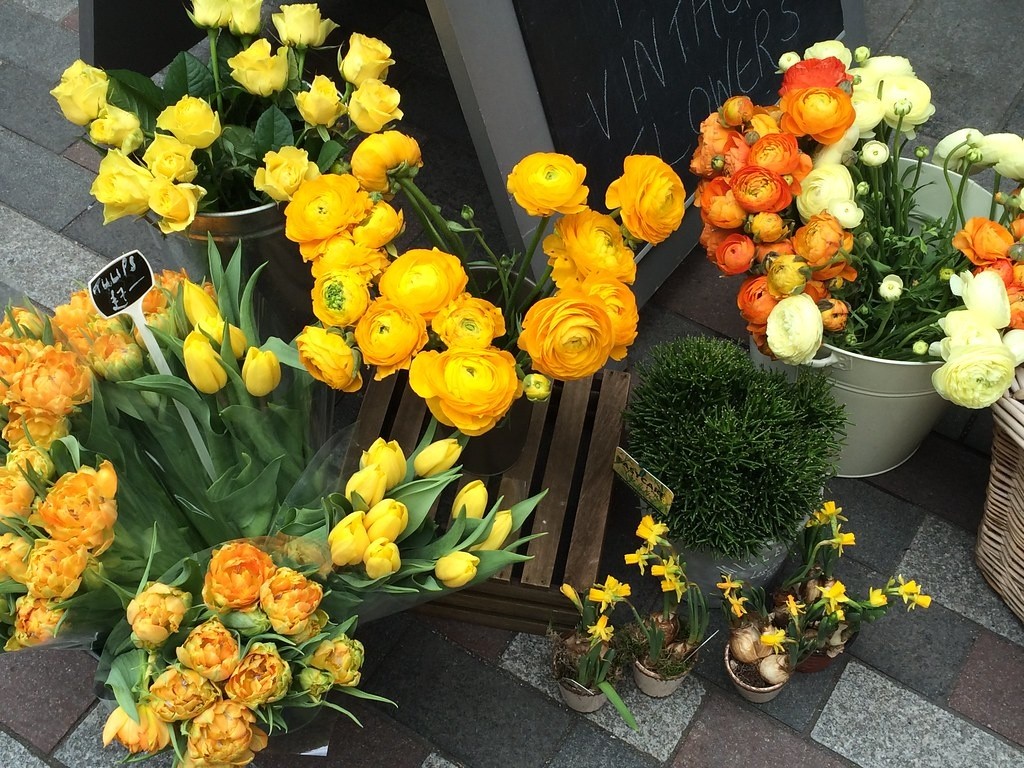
[975,363,1024,623]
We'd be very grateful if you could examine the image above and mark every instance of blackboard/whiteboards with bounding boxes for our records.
[79,1,870,313]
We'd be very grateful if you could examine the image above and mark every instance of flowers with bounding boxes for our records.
[0,0,1024,768]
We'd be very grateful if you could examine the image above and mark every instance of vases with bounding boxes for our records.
[639,477,824,610]
[557,681,609,715]
[725,643,789,705]
[974,363,1024,624]
[633,659,695,699]
[748,154,1014,478]
[797,629,859,675]
[144,196,319,344]
